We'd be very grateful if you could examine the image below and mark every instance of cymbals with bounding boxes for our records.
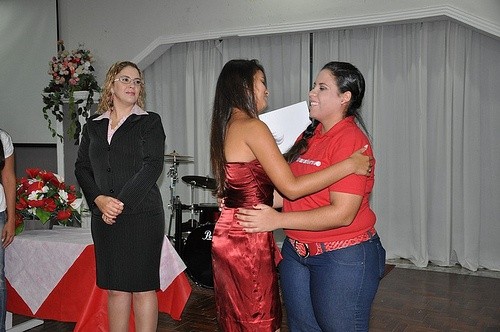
[163,153,194,158]
[181,175,216,190]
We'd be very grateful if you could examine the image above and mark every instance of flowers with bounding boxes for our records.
[16,168,77,230]
[43,40,102,145]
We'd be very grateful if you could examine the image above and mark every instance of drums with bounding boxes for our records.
[199,202,221,225]
[184,223,218,289]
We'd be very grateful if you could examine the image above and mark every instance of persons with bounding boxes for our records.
[208,60,374,332]
[234,61,386,332]
[0,128,17,332]
[74,60,166,332]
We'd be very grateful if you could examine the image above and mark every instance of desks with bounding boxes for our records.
[5,226,193,332]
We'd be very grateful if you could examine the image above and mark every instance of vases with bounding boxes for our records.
[24,219,55,229]
[44,91,101,105]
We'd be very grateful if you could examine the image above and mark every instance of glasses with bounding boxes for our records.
[114,77,142,86]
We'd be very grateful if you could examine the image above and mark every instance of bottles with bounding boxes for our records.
[79,193,91,234]
